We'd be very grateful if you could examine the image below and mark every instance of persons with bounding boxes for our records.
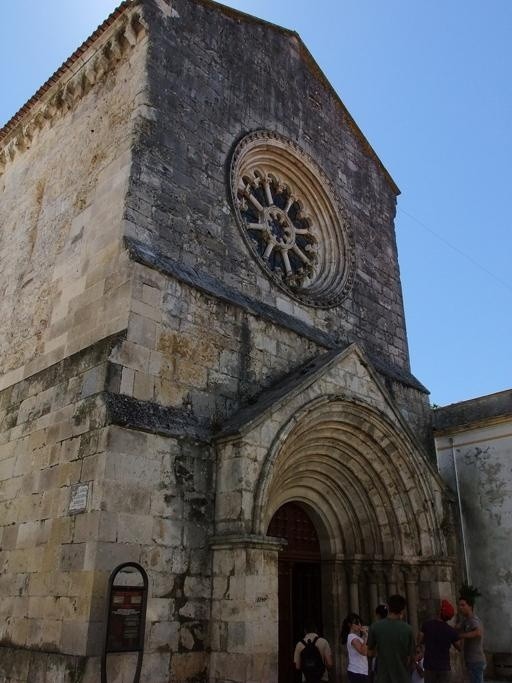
[412,645,425,683]
[455,597,488,683]
[417,600,461,683]
[367,594,416,683]
[293,622,333,682]
[339,613,371,683]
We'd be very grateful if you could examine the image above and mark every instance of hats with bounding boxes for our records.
[442,599,455,618]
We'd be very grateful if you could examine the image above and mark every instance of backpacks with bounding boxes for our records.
[298,635,324,683]
